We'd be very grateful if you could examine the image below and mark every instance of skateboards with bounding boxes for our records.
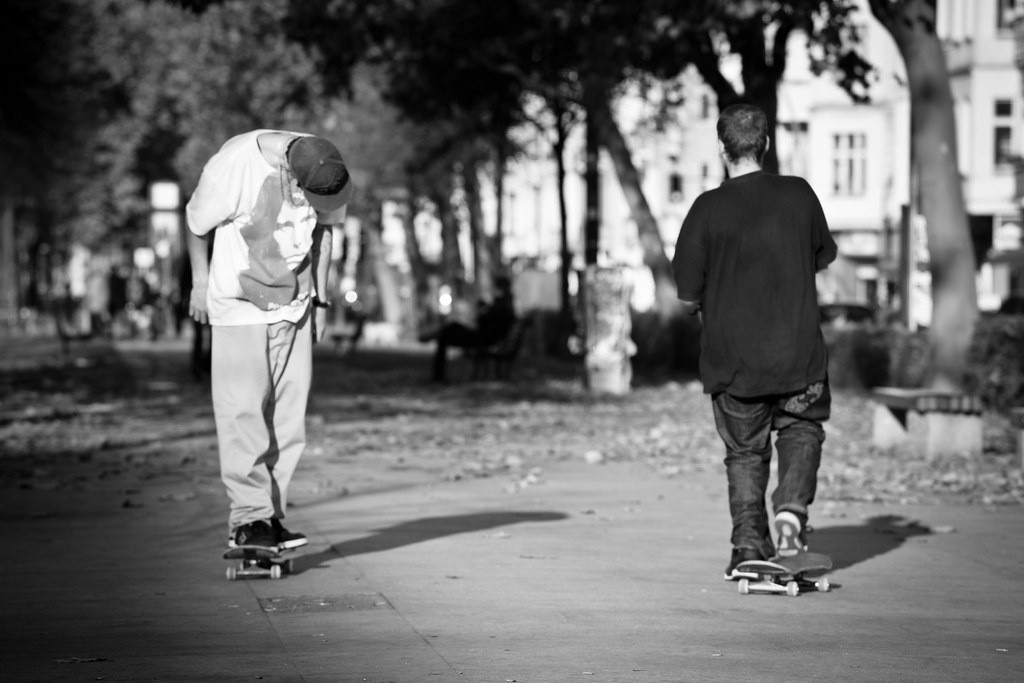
[222,544,297,581]
[732,551,834,598]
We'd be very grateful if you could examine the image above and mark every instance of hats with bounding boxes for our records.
[289,136,352,212]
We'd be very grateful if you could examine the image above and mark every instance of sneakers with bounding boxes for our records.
[722,547,761,579]
[230,519,308,549]
[233,521,280,552]
[774,509,812,557]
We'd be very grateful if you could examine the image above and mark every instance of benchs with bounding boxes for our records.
[872,386,986,458]
[54,312,98,358]
[462,316,533,382]
[330,316,367,351]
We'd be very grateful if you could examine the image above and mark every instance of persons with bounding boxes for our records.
[416,276,516,385]
[186,130,354,552]
[671,101,839,575]
[238,177,317,313]
